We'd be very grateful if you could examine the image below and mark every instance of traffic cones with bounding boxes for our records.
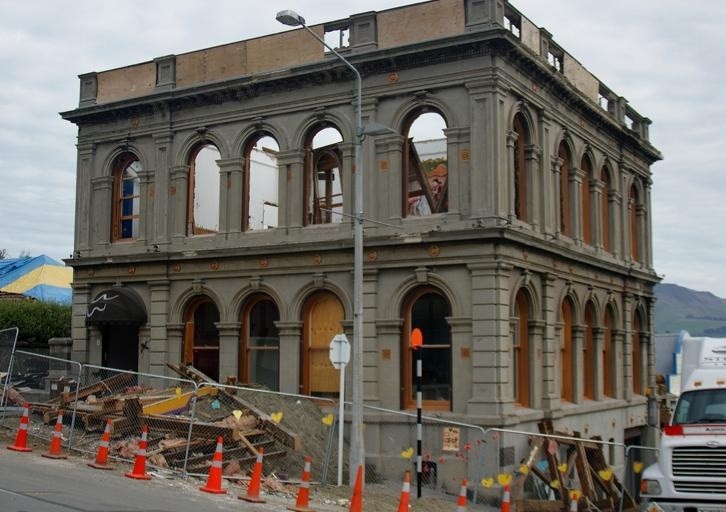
[287,455,318,512]
[41,410,67,459]
[396,471,412,512]
[349,464,364,512]
[86,419,113,470]
[199,436,228,494]
[238,447,267,503]
[455,479,578,512]
[124,425,152,481]
[6,403,33,452]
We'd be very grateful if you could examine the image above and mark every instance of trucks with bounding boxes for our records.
[637,332,725,512]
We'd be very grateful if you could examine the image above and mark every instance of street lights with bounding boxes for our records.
[277,8,366,491]
